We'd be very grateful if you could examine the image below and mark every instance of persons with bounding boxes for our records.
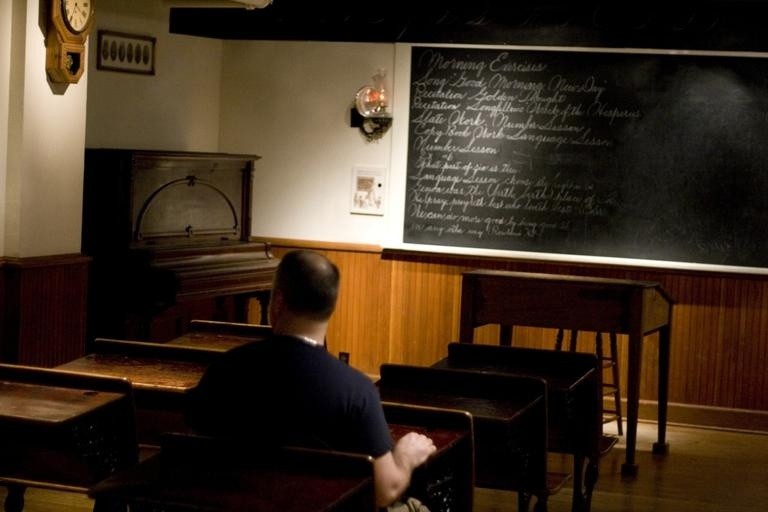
[195,249,436,512]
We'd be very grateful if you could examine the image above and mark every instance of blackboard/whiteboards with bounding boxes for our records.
[400,43,768,277]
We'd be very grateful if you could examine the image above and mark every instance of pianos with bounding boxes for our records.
[86,149,279,337]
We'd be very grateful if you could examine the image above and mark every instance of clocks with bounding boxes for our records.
[39,0,94,84]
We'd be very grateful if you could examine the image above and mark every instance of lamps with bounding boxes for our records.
[349,67,392,137]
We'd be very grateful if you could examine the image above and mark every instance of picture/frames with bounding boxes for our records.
[96,29,157,78]
[349,166,387,217]
